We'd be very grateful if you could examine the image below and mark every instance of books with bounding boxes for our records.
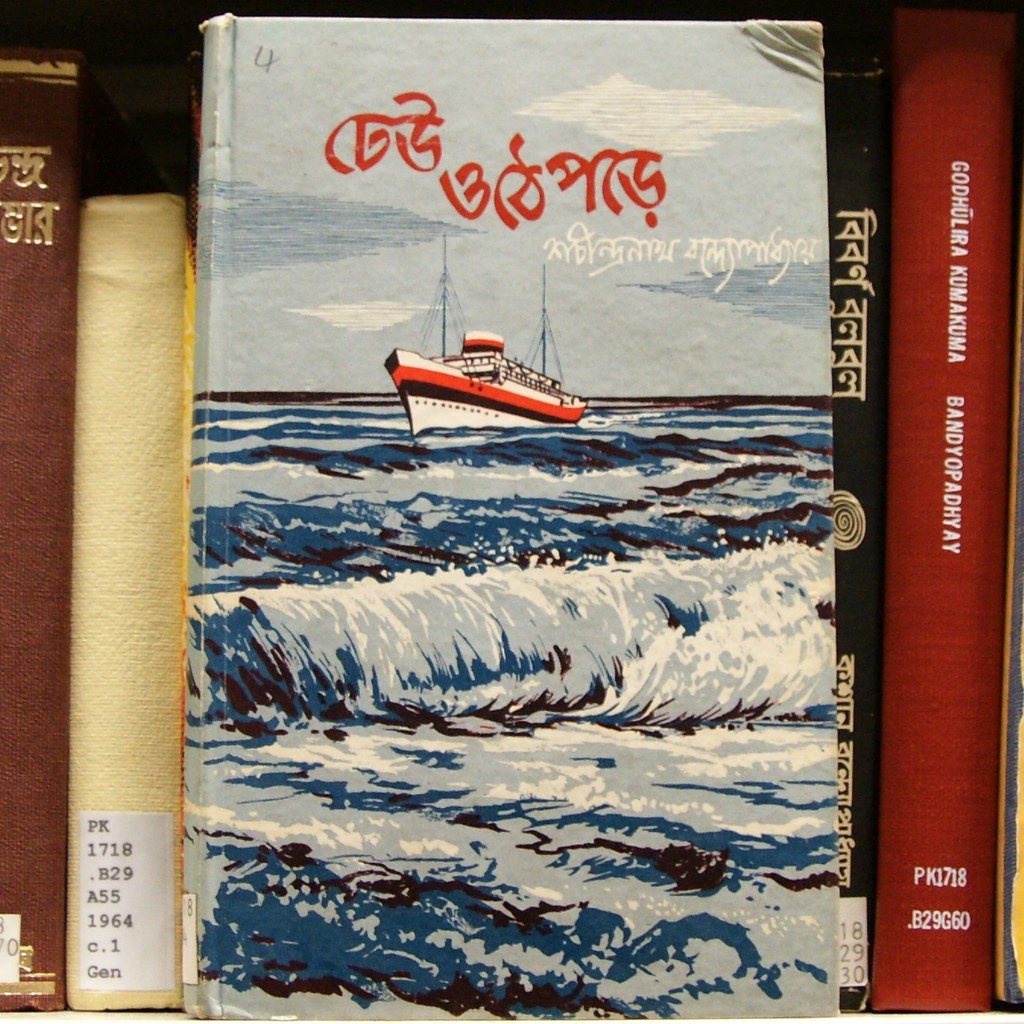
[0,5,1024,1021]
